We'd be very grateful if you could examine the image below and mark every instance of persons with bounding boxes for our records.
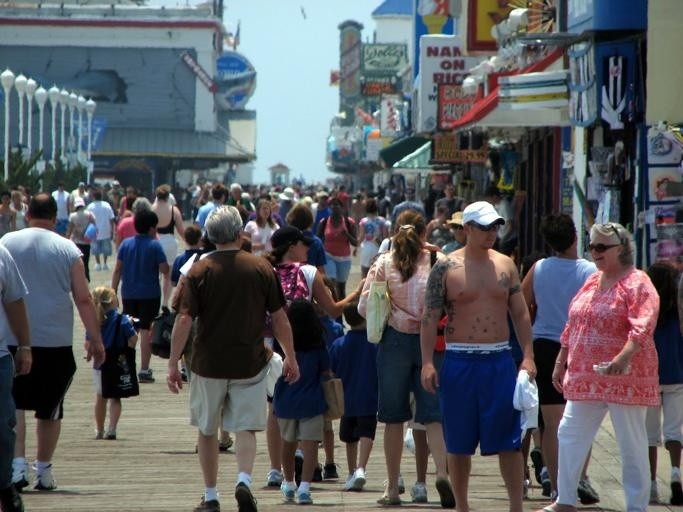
[2,182,682,512]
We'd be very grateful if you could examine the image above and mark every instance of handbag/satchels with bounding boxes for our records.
[320,377,345,421]
[150,312,184,360]
[365,280,392,344]
[81,223,98,244]
[100,347,139,400]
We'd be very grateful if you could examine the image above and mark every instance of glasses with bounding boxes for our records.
[588,243,622,253]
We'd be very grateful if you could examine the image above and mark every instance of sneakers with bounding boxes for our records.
[409,483,428,503]
[1,484,23,512]
[31,463,57,491]
[669,471,683,505]
[93,428,117,440]
[650,489,659,502]
[524,447,559,504]
[381,476,405,494]
[10,462,29,488]
[192,495,220,512]
[93,264,109,271]
[234,481,257,512]
[267,469,284,487]
[217,437,233,451]
[577,474,601,506]
[139,371,157,384]
[435,477,455,509]
[280,480,296,500]
[297,489,314,504]
[376,492,401,506]
[293,449,368,492]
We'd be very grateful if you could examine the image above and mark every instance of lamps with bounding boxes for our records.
[488,6,587,69]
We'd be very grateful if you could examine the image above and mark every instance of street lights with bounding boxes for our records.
[0,66,97,182]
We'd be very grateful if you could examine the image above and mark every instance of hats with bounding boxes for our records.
[461,200,506,227]
[446,211,463,226]
[280,187,298,201]
[317,191,329,198]
[269,226,315,248]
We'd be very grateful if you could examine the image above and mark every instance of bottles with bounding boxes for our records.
[591,360,632,375]
